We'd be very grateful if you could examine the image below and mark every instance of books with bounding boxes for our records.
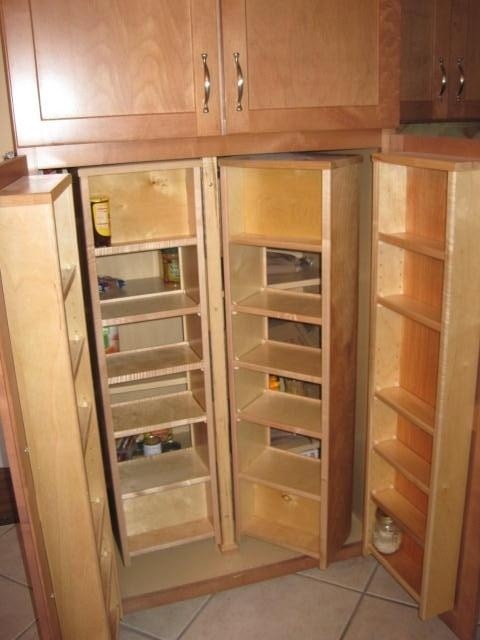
[265,250,302,276]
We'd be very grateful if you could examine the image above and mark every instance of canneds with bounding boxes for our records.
[131,434,144,458]
[143,435,162,456]
[103,326,120,354]
[160,247,180,289]
[374,507,401,553]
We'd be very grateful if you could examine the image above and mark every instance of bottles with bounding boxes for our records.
[371,511,405,557]
